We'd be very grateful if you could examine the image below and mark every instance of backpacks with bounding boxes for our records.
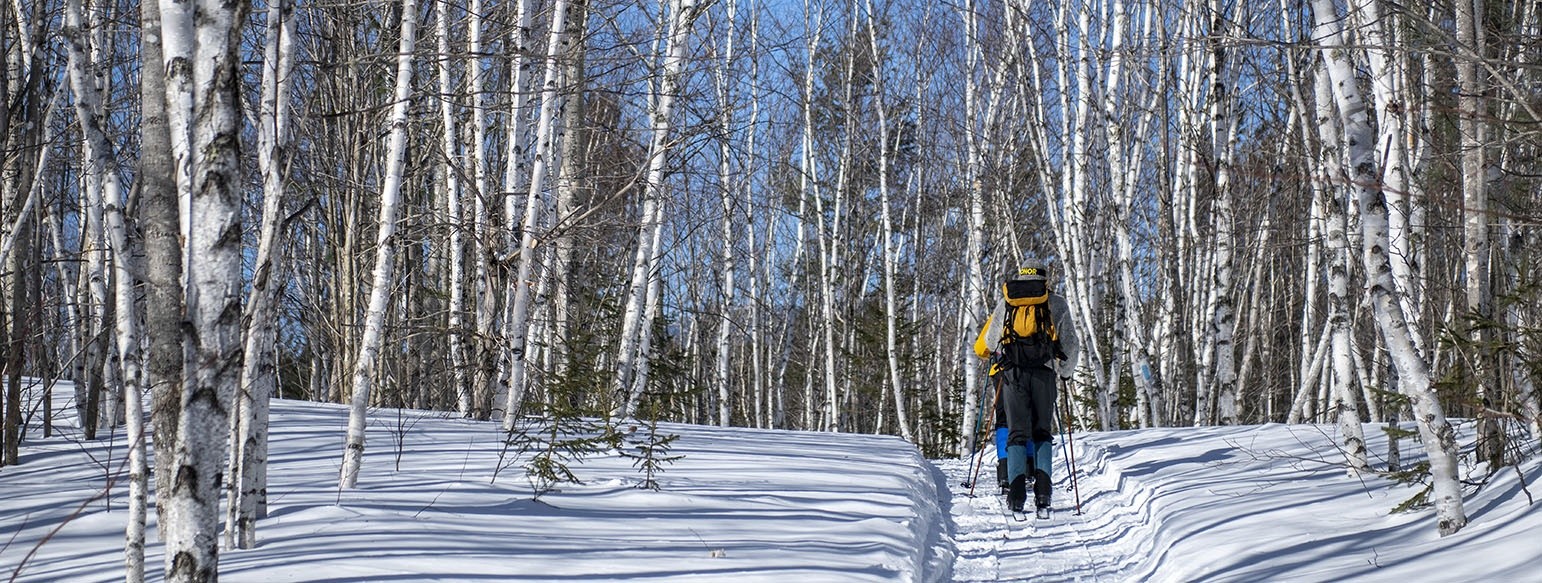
[1004,280,1054,369]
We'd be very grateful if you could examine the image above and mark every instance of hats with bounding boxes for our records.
[1022,260,1046,278]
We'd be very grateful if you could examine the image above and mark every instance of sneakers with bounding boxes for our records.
[1034,494,1052,506]
[1007,498,1024,511]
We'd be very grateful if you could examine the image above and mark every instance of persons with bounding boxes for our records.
[975,314,1035,494]
[986,259,1081,513]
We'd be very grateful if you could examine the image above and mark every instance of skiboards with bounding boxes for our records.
[1006,496,1050,521]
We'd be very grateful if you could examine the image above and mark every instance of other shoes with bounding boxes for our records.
[1026,475,1036,491]
[998,478,1011,494]
